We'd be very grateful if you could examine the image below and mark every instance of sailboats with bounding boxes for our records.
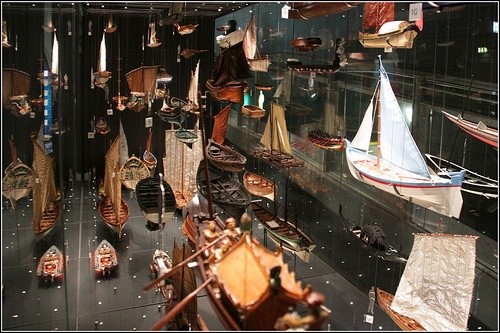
[147,22,161,48]
[377,233,478,332]
[206,19,250,104]
[358,2,424,49]
[3,141,23,177]
[37,33,64,90]
[252,102,306,171]
[30,125,61,241]
[104,15,116,34]
[181,60,202,115]
[342,55,464,220]
[160,238,210,331]
[143,132,157,169]
[94,37,111,87]
[242,14,269,73]
[98,124,129,241]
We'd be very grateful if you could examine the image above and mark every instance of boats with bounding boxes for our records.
[197,160,251,222]
[174,23,198,34]
[205,138,247,172]
[51,118,68,136]
[9,94,31,118]
[244,171,278,201]
[3,157,32,210]
[36,245,65,287]
[92,238,117,279]
[150,248,177,300]
[290,127,345,155]
[120,154,151,194]
[242,104,266,118]
[424,152,498,215]
[152,68,174,84]
[91,114,110,136]
[135,176,174,225]
[157,97,189,125]
[144,212,336,331]
[289,36,322,52]
[339,206,410,265]
[128,91,146,114]
[286,37,347,74]
[32,95,44,115]
[252,202,316,254]
[442,109,497,149]
